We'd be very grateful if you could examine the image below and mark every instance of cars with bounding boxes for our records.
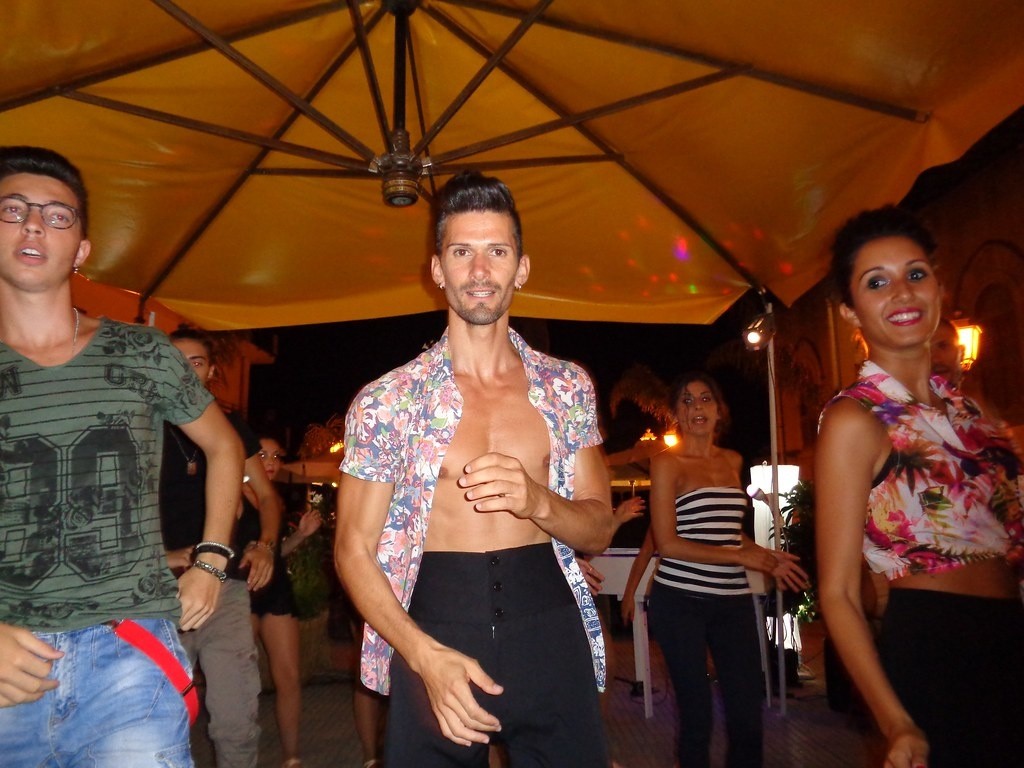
[585,479,659,634]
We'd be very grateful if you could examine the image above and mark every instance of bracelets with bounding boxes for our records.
[191,541,234,582]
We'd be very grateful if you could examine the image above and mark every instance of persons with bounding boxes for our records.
[232,429,323,768]
[353,661,381,768]
[575,497,646,597]
[0,147,246,768]
[159,329,281,768]
[931,320,993,415]
[813,206,1024,768]
[330,165,615,767]
[649,372,808,768]
[621,526,660,627]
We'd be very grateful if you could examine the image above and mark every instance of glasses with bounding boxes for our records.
[0,196,86,237]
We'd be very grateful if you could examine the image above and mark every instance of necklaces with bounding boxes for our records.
[168,425,198,475]
[70,307,79,357]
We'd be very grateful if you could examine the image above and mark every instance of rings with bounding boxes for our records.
[587,567,593,573]
